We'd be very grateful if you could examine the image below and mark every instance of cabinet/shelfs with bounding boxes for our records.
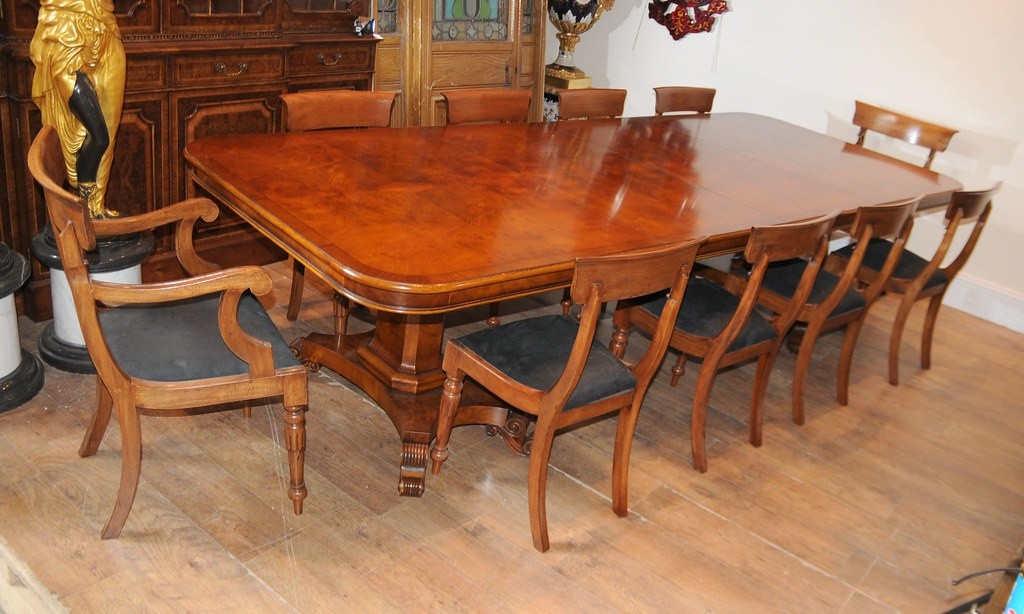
[0,0,383,321]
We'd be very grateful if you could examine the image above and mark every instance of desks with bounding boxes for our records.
[183,111,966,497]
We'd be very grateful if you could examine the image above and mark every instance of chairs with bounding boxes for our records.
[25,87,1002,553]
